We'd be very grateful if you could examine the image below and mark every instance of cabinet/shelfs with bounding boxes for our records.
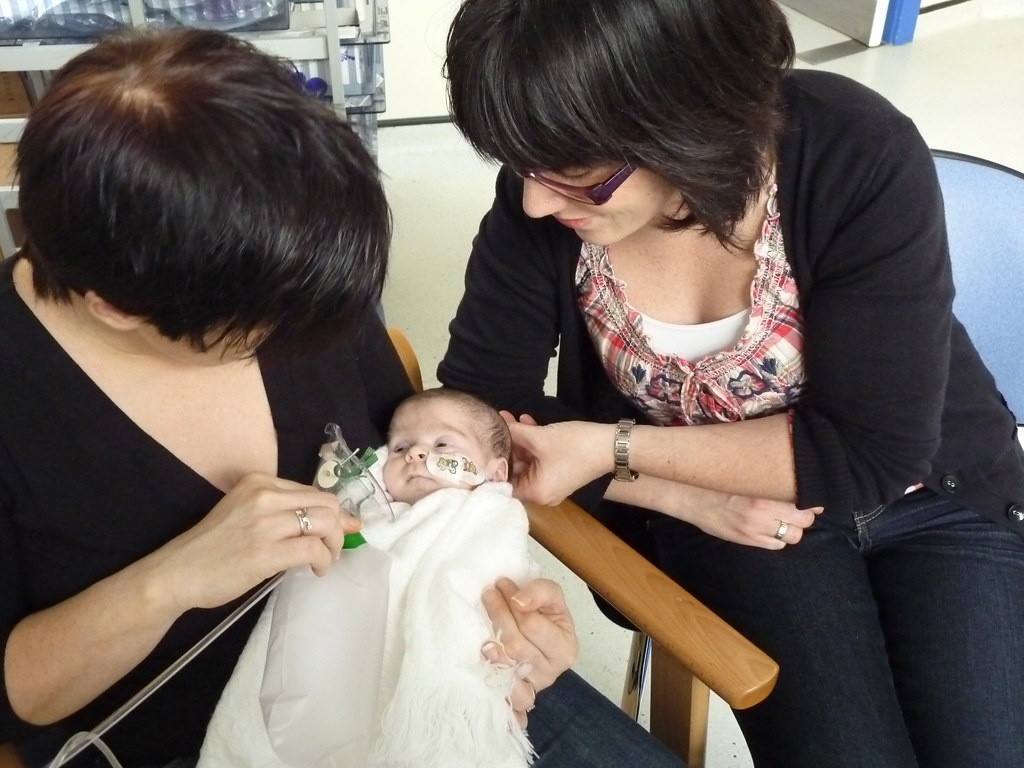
[1,0,391,259]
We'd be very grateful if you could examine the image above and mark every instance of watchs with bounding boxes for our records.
[614,418,639,483]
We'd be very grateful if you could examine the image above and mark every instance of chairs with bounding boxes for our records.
[619,149,1024,726]
[0,326,782,768]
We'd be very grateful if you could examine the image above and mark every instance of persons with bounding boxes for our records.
[435,0,1024,768]
[0,25,581,768]
[195,386,528,768]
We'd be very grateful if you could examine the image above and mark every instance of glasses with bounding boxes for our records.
[509,155,642,205]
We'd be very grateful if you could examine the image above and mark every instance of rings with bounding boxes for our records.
[294,507,314,531]
[775,522,788,539]
